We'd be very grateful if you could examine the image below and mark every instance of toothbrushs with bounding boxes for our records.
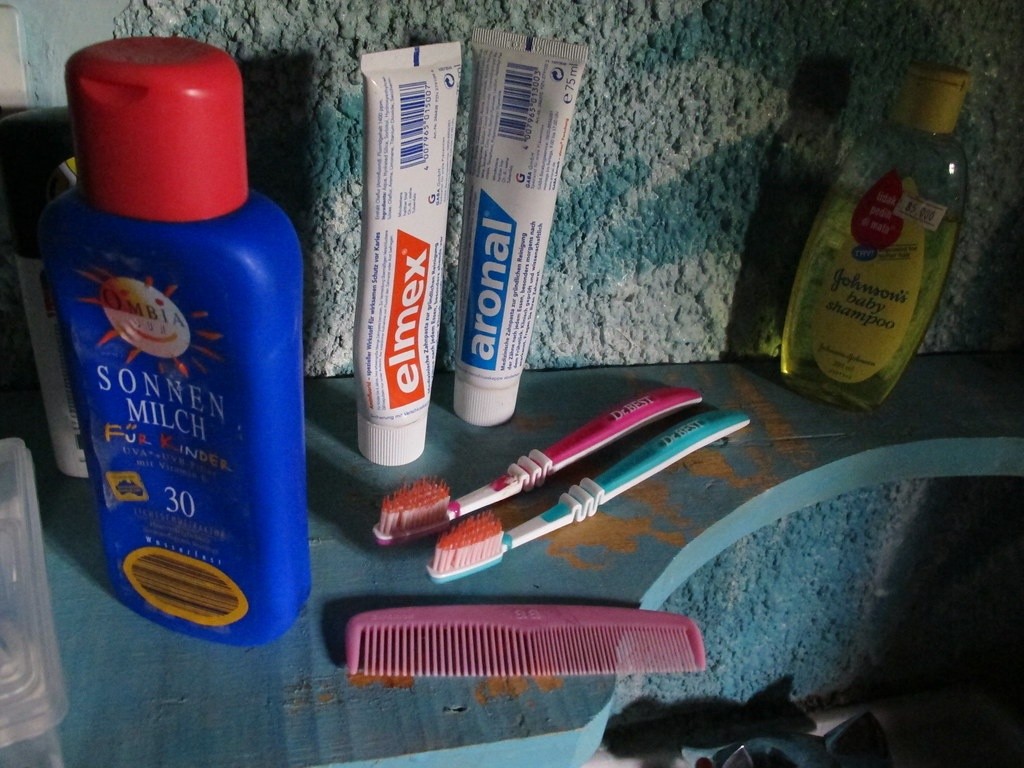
[368,384,707,549]
[422,405,753,586]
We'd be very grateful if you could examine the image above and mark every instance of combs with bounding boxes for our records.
[344,604,706,676]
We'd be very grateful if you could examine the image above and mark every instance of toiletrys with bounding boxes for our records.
[451,26,593,430]
[776,57,975,415]
[350,38,465,467]
[48,35,315,648]
